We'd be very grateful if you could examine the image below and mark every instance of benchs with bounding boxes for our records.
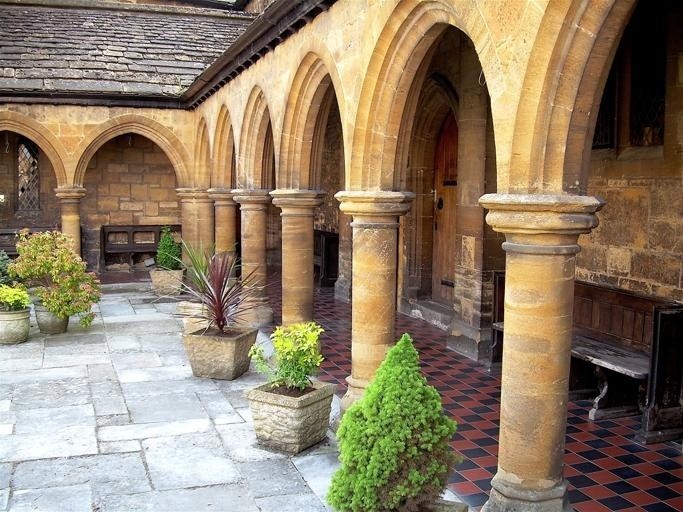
[488,269,682,444]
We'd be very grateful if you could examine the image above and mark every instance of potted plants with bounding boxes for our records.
[0,226,102,345]
